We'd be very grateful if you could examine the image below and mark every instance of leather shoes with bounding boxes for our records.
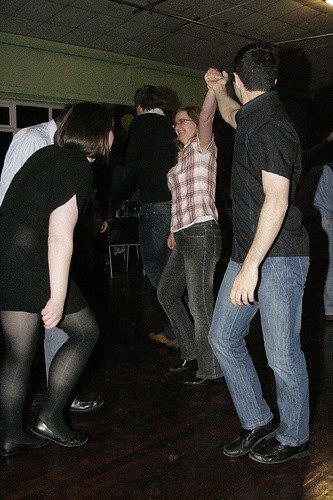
[169,359,192,372]
[1,434,47,456]
[183,375,210,385]
[31,417,88,447]
[248,435,311,464]
[222,416,281,457]
[71,393,104,413]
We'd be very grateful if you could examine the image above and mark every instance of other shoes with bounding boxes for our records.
[150,333,178,346]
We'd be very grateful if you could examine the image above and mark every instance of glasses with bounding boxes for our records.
[172,119,193,129]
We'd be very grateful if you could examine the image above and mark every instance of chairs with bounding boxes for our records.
[104,216,146,279]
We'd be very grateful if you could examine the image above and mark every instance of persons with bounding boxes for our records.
[157,68,222,384]
[314,165,333,320]
[0,102,76,203]
[0,100,115,457]
[205,42,310,464]
[102,85,181,345]
